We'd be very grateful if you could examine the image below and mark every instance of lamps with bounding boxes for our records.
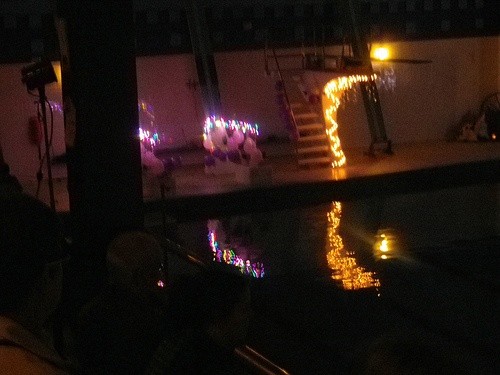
[21,58,62,212]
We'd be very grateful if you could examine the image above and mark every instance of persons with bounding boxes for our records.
[0,192,73,374]
[72,231,234,375]
[60,353,62,355]
